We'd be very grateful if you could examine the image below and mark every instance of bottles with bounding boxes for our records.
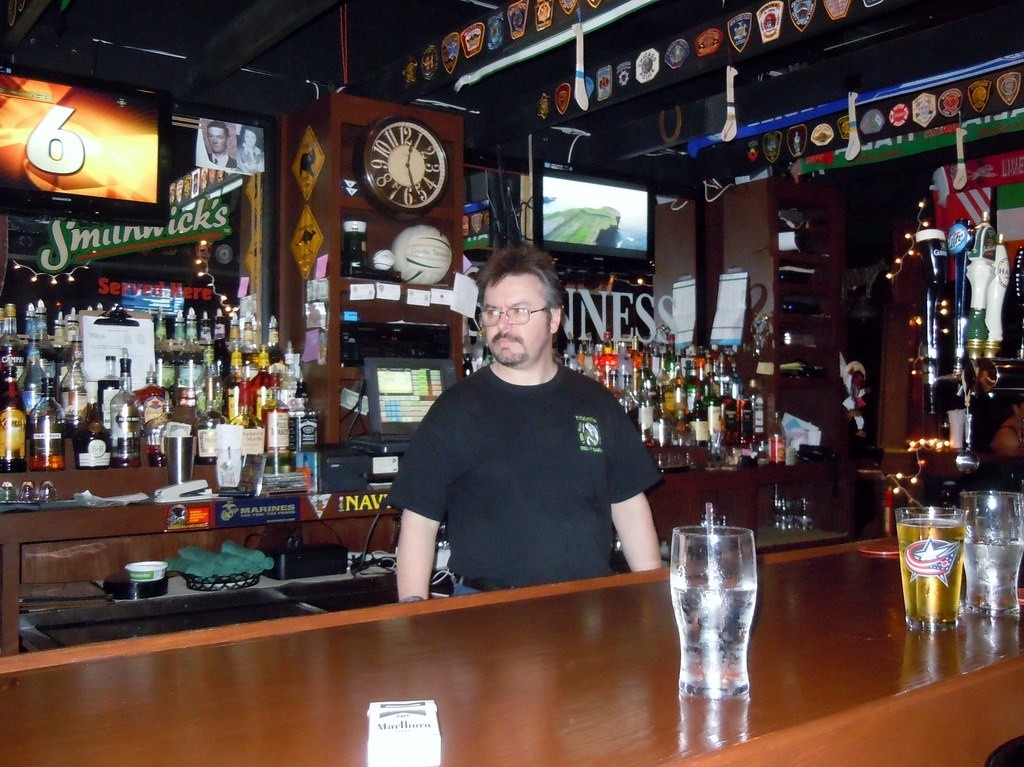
[462,329,787,469]
[0,300,319,501]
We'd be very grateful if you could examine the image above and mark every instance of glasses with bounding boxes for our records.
[478,307,547,326]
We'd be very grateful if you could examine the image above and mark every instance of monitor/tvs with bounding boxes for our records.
[365,356,456,443]
[531,160,657,276]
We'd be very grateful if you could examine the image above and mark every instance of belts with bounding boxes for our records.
[452,575,519,591]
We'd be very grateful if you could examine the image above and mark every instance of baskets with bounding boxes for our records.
[179,570,262,592]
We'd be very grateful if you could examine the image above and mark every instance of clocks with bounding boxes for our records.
[355,114,449,221]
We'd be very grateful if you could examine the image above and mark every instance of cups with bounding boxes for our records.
[124,560,169,582]
[342,220,369,279]
[895,506,968,632]
[669,524,759,699]
[960,490,1024,617]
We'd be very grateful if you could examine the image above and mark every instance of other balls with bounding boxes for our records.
[392,225,453,286]
[373,248,396,270]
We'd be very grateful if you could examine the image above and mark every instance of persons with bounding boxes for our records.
[207,121,264,174]
[384,247,663,603]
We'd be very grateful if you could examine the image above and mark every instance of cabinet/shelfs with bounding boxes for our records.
[723,177,848,455]
[277,93,466,442]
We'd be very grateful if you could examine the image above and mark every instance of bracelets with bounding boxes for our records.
[400,596,423,602]
